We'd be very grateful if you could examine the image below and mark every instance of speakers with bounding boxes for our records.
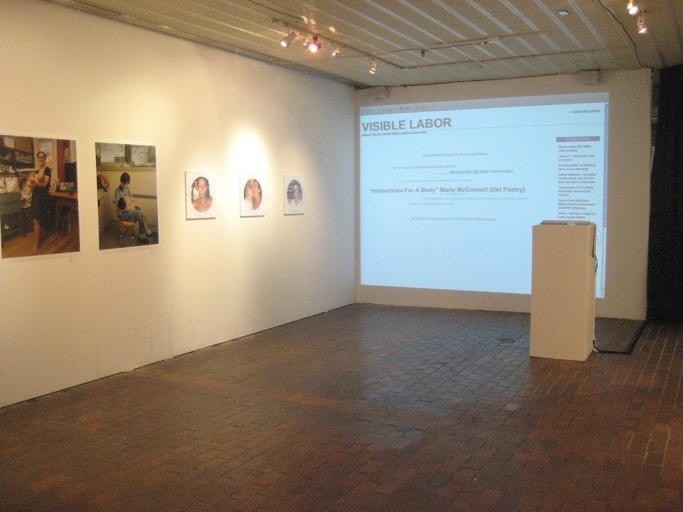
[578,70,601,85]
[368,86,388,100]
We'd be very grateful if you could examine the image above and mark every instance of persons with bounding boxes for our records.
[192,177,212,212]
[244,179,262,210]
[113,172,153,236]
[289,184,302,209]
[23,151,51,252]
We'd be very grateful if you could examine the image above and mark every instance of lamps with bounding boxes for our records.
[627,0,648,34]
[281,31,321,53]
[369,61,376,74]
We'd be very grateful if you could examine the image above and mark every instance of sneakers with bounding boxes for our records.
[137,231,158,244]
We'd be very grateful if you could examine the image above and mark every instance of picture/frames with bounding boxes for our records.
[184,171,306,218]
